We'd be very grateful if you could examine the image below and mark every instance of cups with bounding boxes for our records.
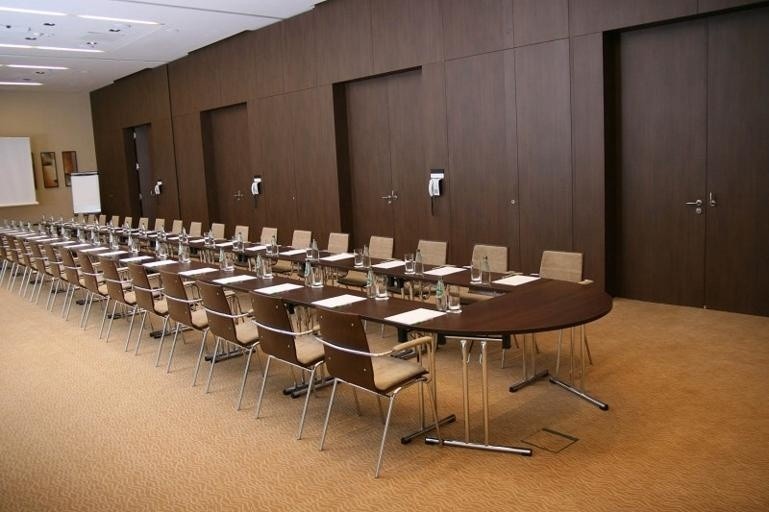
[63,221,279,281]
[311,266,323,285]
[353,248,363,266]
[447,283,462,310]
[306,250,312,259]
[375,274,388,298]
[404,253,414,271]
[482,254,491,284]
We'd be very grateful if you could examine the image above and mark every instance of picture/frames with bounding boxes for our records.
[62,151,77,186]
[40,152,59,188]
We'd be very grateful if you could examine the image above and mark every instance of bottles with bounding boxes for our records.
[312,239,320,261]
[366,267,376,299]
[304,259,313,286]
[3,214,323,288]
[363,243,371,268]
[469,244,483,283]
[434,275,448,309]
[415,250,424,275]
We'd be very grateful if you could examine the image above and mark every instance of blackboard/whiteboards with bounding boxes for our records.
[71,172,101,214]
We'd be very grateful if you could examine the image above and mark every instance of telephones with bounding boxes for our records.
[155,185,160,195]
[428,178,441,198]
[251,182,259,195]
